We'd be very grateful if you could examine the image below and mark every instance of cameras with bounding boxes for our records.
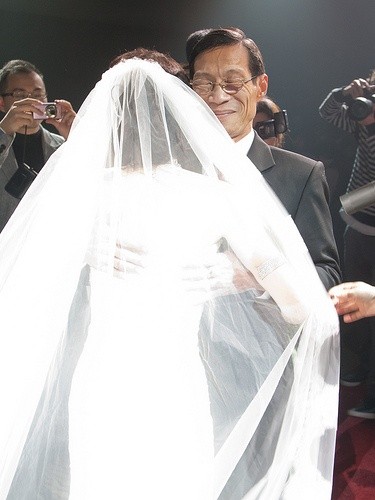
[254,110,289,139]
[349,93,375,120]
[5,163,37,199]
[32,102,61,120]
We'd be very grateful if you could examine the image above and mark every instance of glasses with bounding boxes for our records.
[1,89,48,100]
[190,72,260,96]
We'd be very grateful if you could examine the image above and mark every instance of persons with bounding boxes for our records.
[0,49,342,499]
[0,60,77,234]
[328,282,375,323]
[318,67,375,419]
[184,27,342,291]
[252,98,289,148]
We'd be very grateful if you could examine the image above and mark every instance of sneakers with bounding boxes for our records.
[348,394,375,419]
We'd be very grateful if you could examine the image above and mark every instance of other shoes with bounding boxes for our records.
[337,371,364,388]
